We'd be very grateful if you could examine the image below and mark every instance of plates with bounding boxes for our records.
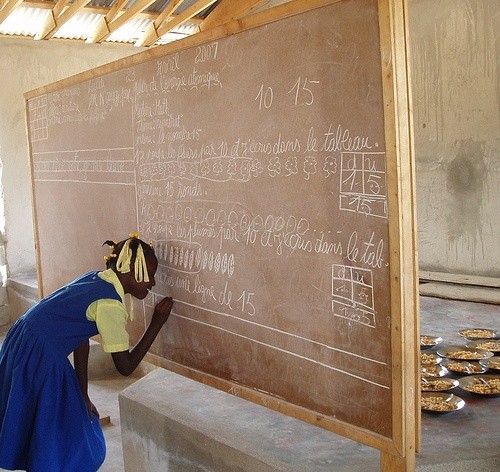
[457,375,500,397]
[437,346,500,373]
[457,328,495,339]
[465,340,500,354]
[420,335,443,349]
[421,365,448,378]
[421,353,443,365]
[421,378,459,391]
[421,392,465,414]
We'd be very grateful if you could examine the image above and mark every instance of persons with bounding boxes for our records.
[0,232,175,472]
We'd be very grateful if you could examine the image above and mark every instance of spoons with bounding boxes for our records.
[462,361,472,374]
[422,378,435,386]
[479,377,496,389]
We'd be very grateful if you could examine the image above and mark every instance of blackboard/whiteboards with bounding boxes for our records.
[22,1,422,456]
